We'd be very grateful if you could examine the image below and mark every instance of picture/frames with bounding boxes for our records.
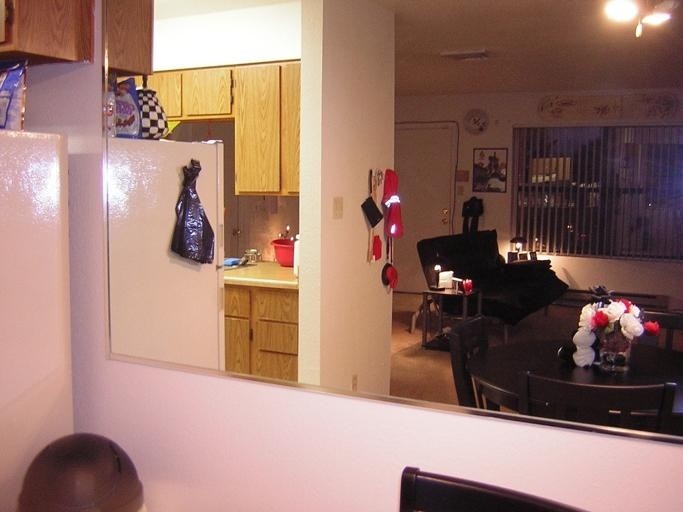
[472,148,508,193]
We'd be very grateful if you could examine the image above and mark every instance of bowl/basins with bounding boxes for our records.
[270,237,294,267]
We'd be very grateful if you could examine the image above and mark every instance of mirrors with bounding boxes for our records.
[98,2,682,445]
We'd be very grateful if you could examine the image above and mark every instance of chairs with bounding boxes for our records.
[449,311,682,432]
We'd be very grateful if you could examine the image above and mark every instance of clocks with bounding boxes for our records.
[464,109,489,134]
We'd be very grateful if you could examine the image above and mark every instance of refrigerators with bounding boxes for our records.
[0,128,75,512]
[105,136,225,371]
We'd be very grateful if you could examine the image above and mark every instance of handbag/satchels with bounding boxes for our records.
[462,196,483,241]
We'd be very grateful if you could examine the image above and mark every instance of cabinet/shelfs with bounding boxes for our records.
[0,0,83,65]
[105,1,155,77]
[142,69,233,120]
[223,284,251,376]
[251,289,298,382]
[232,63,300,194]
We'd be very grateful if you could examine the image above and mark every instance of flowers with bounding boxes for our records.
[570,297,661,368]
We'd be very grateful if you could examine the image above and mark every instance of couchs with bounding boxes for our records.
[409,229,565,337]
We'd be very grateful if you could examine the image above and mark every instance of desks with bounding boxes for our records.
[423,288,483,351]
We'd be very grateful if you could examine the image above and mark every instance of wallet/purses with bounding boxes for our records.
[361,169,383,228]
